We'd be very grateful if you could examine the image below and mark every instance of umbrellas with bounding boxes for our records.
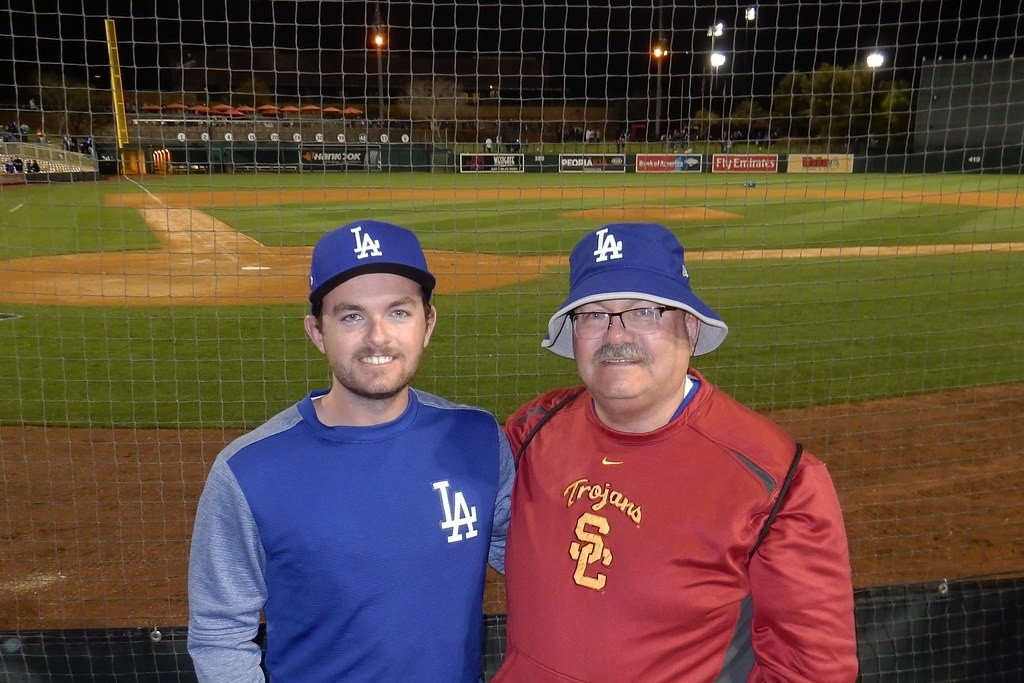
[142,102,363,117]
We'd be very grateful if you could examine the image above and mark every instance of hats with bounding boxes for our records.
[309,217,436,316]
[539,222,730,360]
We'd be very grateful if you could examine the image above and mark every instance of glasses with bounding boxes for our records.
[569,304,678,341]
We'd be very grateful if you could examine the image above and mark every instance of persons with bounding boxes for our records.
[504,223,858,683]
[659,125,779,154]
[558,126,601,143]
[63,134,95,154]
[485,135,521,153]
[0,120,46,145]
[4,155,41,175]
[30,98,38,110]
[618,130,631,153]
[188,221,514,683]
[339,117,401,129]
